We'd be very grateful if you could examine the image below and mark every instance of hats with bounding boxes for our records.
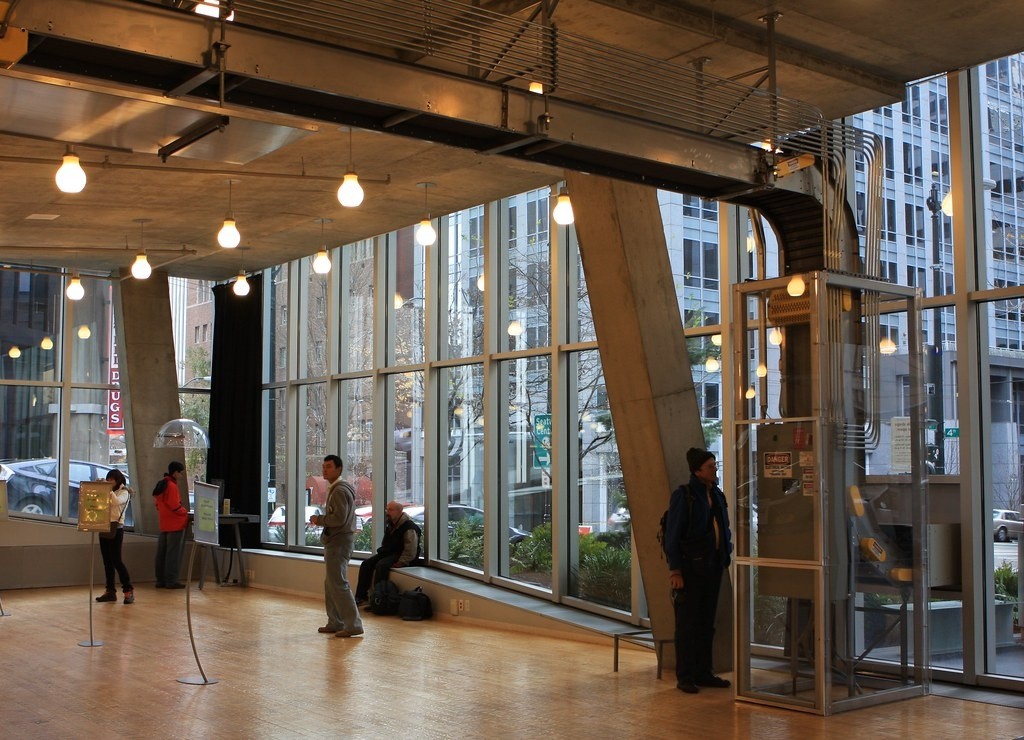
[686,447,715,474]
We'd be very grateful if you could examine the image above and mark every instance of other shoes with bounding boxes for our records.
[355,598,372,612]
[156,582,186,589]
[677,675,731,694]
[318,624,364,637]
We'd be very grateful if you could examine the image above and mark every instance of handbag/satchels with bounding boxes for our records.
[398,586,433,622]
[100,521,118,538]
[371,582,398,616]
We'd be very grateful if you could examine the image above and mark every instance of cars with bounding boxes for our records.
[268,504,325,543]
[608,508,634,533]
[355,503,373,530]
[993,509,1023,542]
[0,457,134,528]
[401,505,533,554]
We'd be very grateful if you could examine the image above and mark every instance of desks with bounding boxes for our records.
[187,512,260,590]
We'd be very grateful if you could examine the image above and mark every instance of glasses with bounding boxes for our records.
[385,508,394,512]
[702,461,719,471]
[180,471,183,475]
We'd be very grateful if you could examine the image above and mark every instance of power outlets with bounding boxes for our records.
[459,599,464,611]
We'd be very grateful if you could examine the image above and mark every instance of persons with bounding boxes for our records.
[355,500,422,611]
[310,455,364,638]
[662,447,735,694]
[95,469,135,604]
[151,460,189,589]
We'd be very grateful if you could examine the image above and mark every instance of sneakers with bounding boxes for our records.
[124,591,135,604]
[95,590,118,602]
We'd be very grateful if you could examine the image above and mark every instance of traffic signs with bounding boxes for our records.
[533,414,552,469]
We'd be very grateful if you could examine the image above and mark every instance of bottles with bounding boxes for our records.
[315,509,319,517]
